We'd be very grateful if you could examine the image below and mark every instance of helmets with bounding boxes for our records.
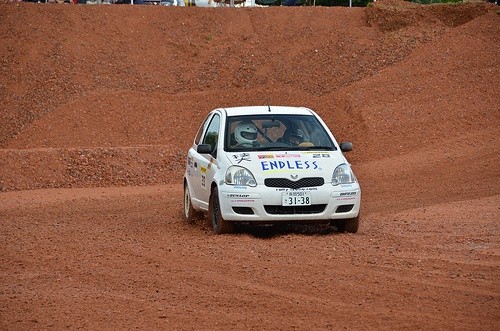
[234,121,258,148]
[283,127,304,146]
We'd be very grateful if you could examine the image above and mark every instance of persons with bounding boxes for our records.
[234,122,260,147]
[283,128,304,146]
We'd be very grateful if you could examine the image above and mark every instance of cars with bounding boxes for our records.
[183,106,361,235]
